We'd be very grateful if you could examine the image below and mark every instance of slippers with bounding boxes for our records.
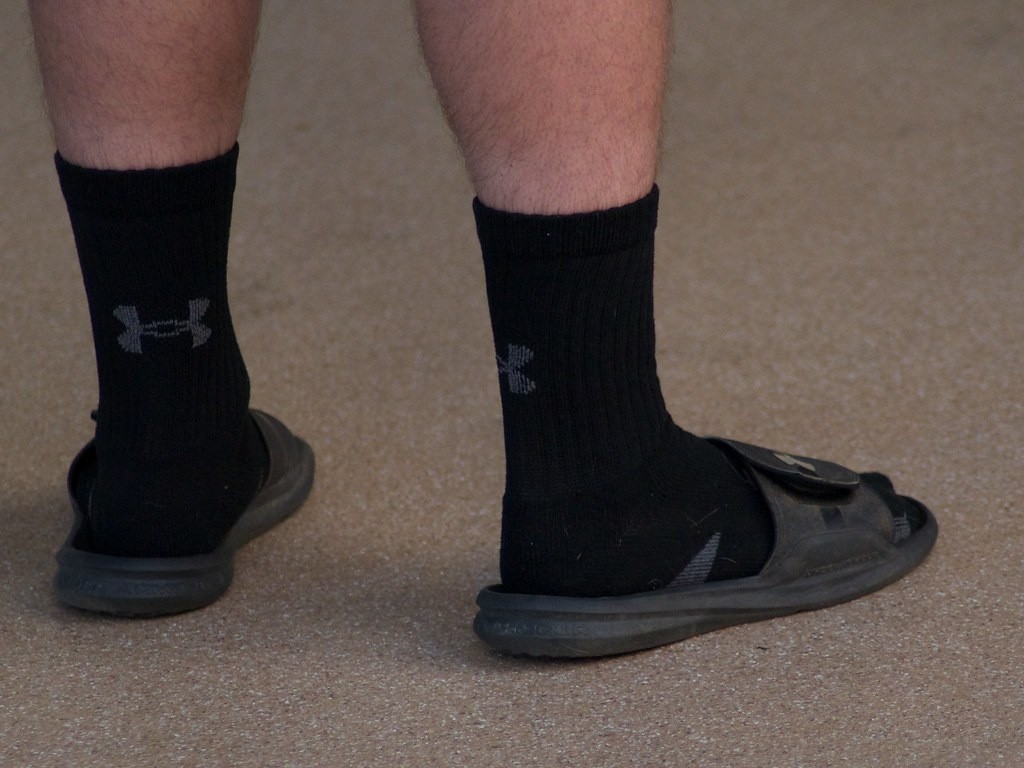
[474,436,942,658]
[53,407,316,618]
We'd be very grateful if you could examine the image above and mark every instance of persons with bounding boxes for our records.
[27,0,942,662]
